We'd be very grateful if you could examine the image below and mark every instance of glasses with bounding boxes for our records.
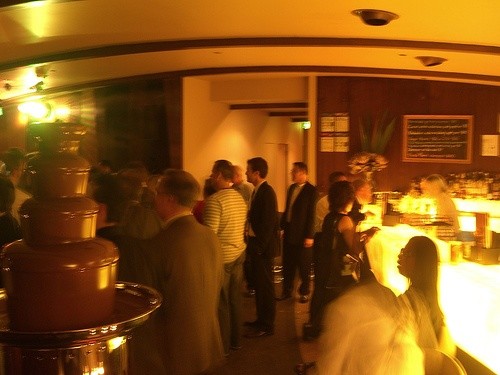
[400,248,410,257]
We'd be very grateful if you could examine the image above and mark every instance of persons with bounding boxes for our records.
[127,169,225,375]
[0,147,281,358]
[276,162,461,375]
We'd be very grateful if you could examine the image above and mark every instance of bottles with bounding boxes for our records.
[449,170,493,199]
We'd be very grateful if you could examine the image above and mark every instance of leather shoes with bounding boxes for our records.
[242,319,258,328]
[242,327,271,338]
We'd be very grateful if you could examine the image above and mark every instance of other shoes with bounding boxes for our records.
[244,284,255,297]
[276,292,293,301]
[299,295,308,303]
